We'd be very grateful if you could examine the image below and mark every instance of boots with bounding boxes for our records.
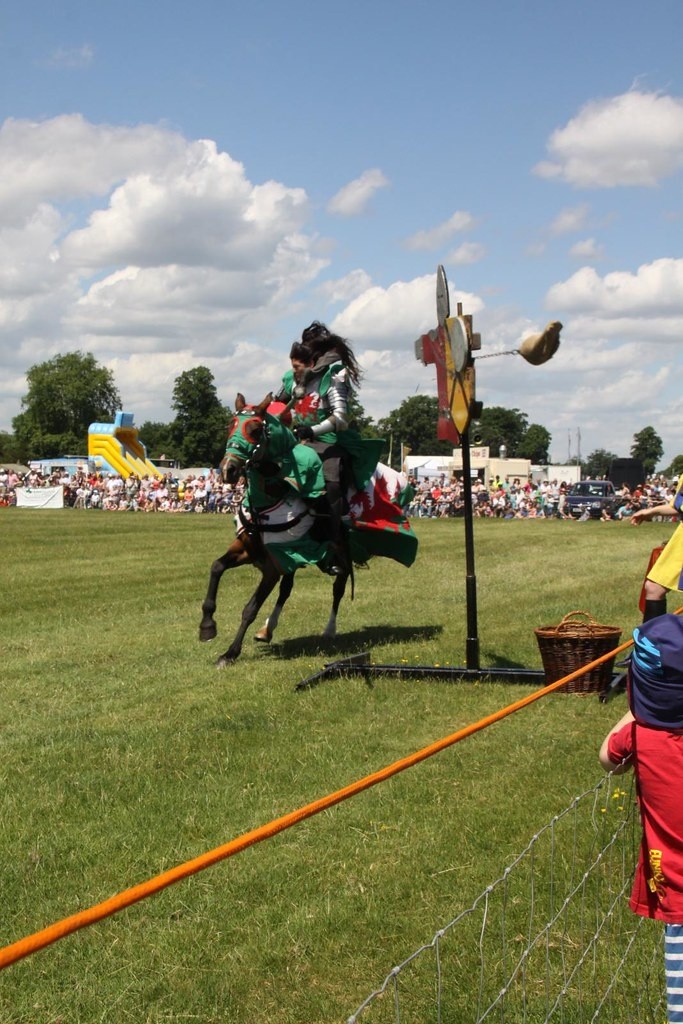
[323,496,347,576]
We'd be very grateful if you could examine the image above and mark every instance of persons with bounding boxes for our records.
[0,458,681,531]
[272,322,365,525]
[597,614,683,1023]
[614,474,682,667]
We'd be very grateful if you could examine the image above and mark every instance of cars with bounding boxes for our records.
[555,481,616,518]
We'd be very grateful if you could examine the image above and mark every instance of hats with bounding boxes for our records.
[632,612,683,728]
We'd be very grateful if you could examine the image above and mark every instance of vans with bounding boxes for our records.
[608,456,645,492]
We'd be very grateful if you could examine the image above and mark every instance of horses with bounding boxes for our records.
[198,392,375,669]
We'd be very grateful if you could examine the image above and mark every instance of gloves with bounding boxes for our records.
[293,423,313,438]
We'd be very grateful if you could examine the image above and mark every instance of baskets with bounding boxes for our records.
[534,608,622,697]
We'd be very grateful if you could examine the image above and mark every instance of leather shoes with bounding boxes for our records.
[615,654,632,668]
